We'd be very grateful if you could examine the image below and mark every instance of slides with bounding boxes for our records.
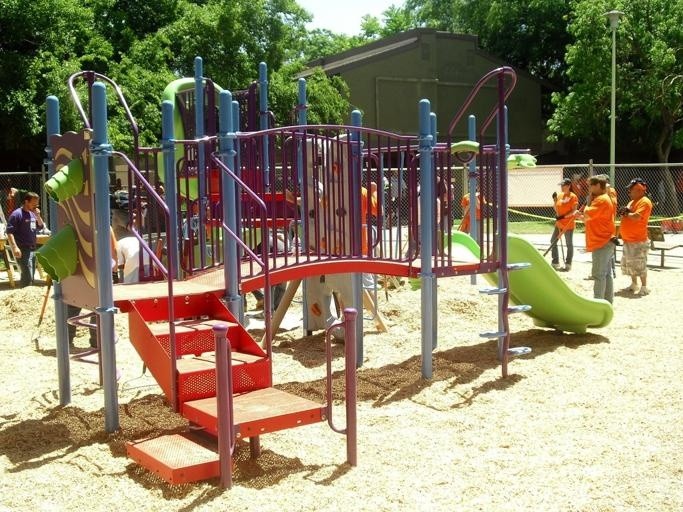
[442,229,613,333]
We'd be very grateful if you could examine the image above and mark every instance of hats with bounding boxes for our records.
[624,178,644,189]
[557,178,572,186]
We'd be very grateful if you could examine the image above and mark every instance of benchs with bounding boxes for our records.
[610,220,681,270]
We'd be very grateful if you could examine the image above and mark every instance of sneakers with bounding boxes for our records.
[638,287,646,296]
[624,285,640,292]
[565,264,571,271]
[552,263,560,270]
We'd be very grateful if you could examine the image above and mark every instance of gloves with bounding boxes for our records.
[555,215,565,221]
[618,206,631,217]
[552,191,557,199]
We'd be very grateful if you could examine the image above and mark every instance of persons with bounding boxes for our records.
[115,231,152,283]
[584,172,616,280]
[66,225,116,353]
[249,229,290,313]
[458,183,488,248]
[571,175,614,305]
[182,205,211,245]
[370,173,453,254]
[550,178,578,271]
[619,178,652,296]
[362,178,377,309]
[144,182,166,232]
[5,192,44,288]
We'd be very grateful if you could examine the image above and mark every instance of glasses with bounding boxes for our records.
[32,202,39,207]
